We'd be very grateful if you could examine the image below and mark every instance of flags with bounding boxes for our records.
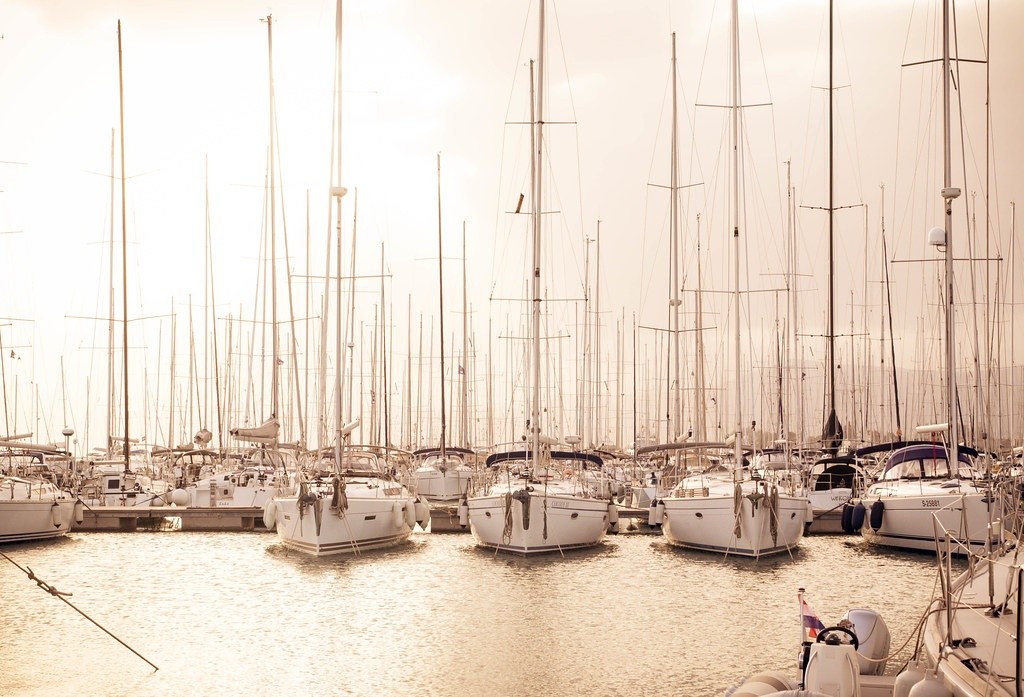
[797,594,830,641]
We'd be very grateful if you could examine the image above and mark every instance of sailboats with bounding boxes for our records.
[1,0,1024,696]
